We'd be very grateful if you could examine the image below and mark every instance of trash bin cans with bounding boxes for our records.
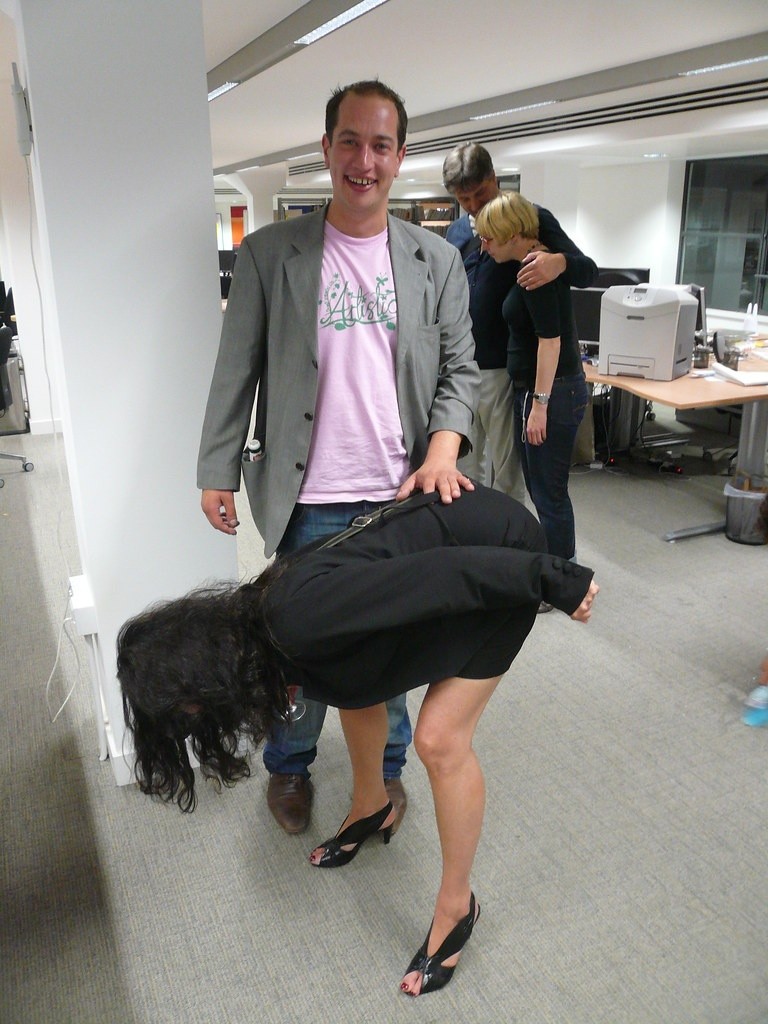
[724,478,768,547]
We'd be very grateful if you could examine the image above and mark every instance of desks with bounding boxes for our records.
[581,329,768,541]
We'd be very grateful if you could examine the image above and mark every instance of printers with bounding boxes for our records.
[597,284,699,382]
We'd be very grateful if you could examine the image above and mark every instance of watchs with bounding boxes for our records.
[534,393,549,404]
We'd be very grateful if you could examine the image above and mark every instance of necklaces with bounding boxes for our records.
[522,243,542,260]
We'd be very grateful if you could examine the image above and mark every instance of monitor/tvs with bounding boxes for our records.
[690,283,706,349]
[568,266,650,358]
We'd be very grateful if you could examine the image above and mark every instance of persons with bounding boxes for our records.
[116,473,598,996]
[441,139,600,506]
[473,189,588,614]
[198,80,484,833]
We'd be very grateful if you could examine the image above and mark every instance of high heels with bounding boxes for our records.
[308,798,395,869]
[399,891,481,997]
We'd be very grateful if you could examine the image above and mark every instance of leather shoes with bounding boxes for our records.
[267,772,314,836]
[386,775,409,836]
